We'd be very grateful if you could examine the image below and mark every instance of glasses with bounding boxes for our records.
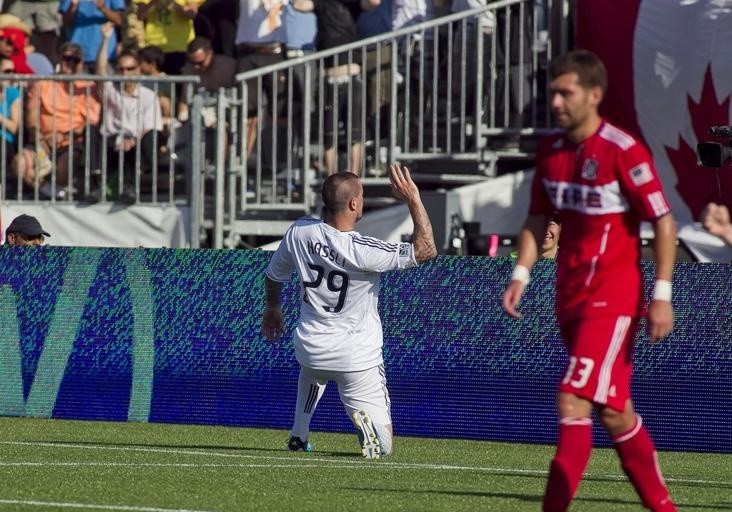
[61,55,80,63]
[189,55,207,65]
[116,66,136,70]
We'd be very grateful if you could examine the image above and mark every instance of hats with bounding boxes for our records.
[6,215,50,237]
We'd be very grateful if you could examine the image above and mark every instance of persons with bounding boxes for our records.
[510,209,564,258]
[699,202,732,255]
[2,213,51,246]
[1,0,567,207]
[501,48,688,512]
[260,161,438,460]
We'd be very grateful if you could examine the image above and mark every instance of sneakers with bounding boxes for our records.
[352,411,381,459]
[122,183,136,203]
[286,436,312,452]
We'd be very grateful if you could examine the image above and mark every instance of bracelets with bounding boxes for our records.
[509,263,532,284]
[652,279,674,303]
